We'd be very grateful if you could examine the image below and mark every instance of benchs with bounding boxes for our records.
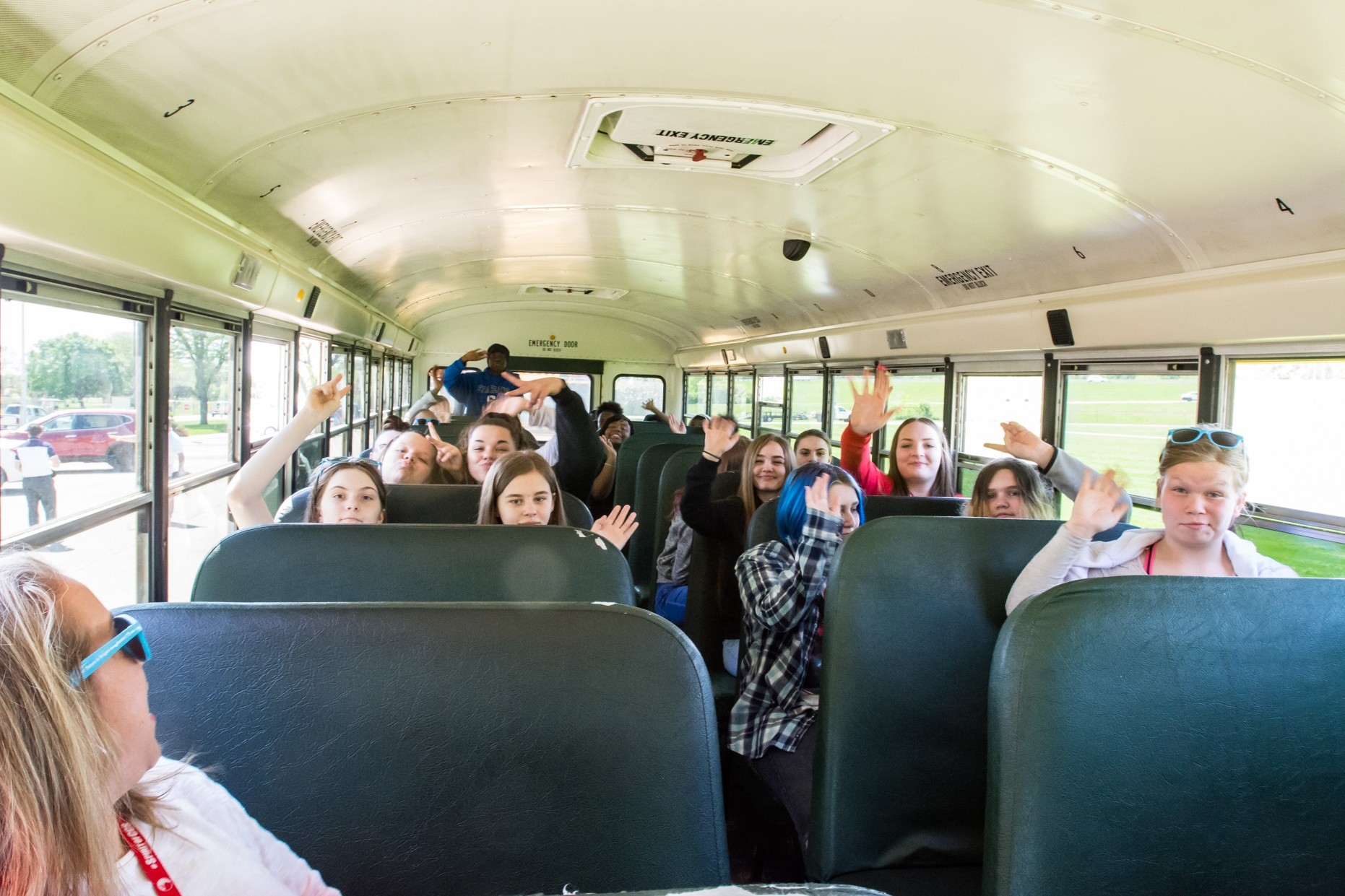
[107,418,1344,896]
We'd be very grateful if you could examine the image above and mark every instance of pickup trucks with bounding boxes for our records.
[812,406,852,422]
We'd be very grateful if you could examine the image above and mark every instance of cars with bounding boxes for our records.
[1,404,50,428]
[1086,375,1110,383]
[0,438,23,486]
[1181,391,1198,401]
[330,404,361,426]
[211,401,230,416]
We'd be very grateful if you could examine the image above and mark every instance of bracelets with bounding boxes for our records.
[702,450,721,461]
[604,463,616,468]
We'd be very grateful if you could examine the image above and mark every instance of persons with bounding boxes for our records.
[225,373,387,530]
[0,549,342,896]
[477,450,638,553]
[367,342,633,489]
[968,421,1132,524]
[641,398,834,676]
[1006,425,1302,616]
[726,462,863,854]
[840,370,965,499]
[15,424,61,526]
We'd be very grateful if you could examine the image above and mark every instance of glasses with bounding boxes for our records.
[1166,428,1244,459]
[319,456,382,478]
[413,418,440,426]
[69,613,153,689]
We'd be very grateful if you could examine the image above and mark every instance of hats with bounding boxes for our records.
[487,343,510,364]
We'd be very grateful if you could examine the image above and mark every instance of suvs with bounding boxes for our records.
[0,408,136,471]
